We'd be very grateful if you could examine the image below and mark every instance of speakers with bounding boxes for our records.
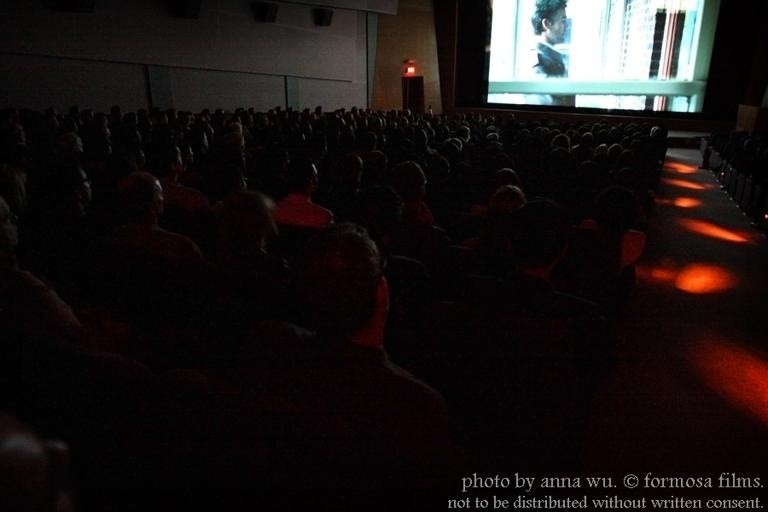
[49,0,97,14]
[313,8,334,26]
[401,75,426,114]
[167,0,202,19]
[249,1,278,23]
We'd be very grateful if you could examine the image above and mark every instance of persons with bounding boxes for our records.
[530,1,576,107]
[0,101,767,510]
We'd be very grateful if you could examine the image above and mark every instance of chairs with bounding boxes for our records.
[0,183,650,511]
